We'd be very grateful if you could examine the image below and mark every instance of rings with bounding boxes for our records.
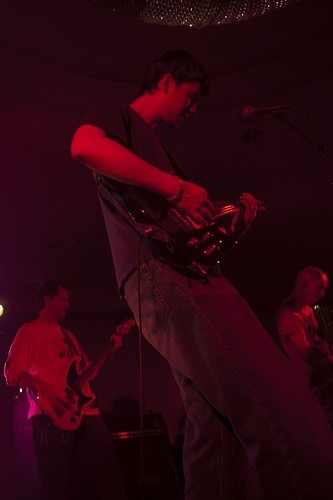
[250,206,256,210]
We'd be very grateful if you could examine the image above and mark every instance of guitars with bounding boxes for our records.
[28,319,135,430]
[123,184,267,266]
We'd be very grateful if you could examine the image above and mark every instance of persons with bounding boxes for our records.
[69,47,333,500]
[275,265,333,385]
[3,280,124,500]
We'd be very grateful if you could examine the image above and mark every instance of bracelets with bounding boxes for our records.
[166,175,185,203]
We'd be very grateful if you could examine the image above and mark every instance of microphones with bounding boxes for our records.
[241,103,296,118]
